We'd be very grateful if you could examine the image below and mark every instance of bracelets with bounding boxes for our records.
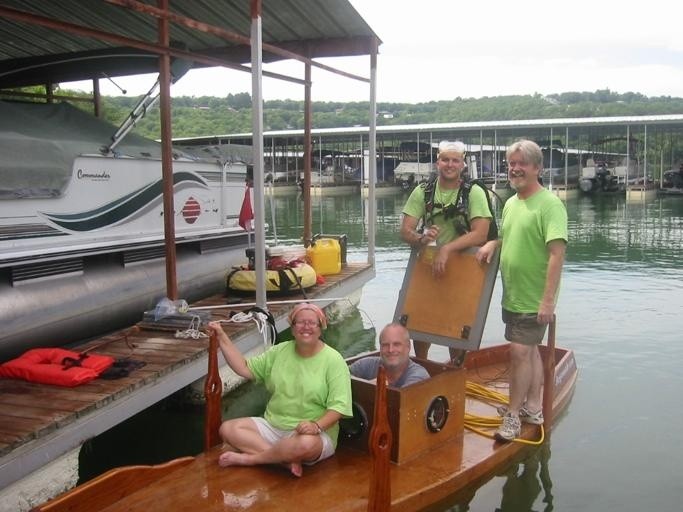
[420,234,425,245]
[308,420,322,434]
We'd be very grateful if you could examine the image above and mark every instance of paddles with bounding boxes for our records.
[105,51,195,152]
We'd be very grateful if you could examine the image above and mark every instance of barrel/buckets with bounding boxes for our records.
[306,238,342,276]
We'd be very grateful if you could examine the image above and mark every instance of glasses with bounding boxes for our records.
[292,319,320,329]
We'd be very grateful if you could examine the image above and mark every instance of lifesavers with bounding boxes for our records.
[224,261,317,297]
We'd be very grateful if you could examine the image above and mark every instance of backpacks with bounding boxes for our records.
[424,172,499,241]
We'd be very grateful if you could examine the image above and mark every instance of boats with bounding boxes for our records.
[31,340,580,512]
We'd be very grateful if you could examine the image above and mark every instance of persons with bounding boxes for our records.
[476,139,569,442]
[346,319,432,388]
[207,302,353,477]
[596,162,611,192]
[398,137,498,367]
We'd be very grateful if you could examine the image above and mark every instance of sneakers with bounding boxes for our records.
[493,411,521,441]
[496,405,544,425]
